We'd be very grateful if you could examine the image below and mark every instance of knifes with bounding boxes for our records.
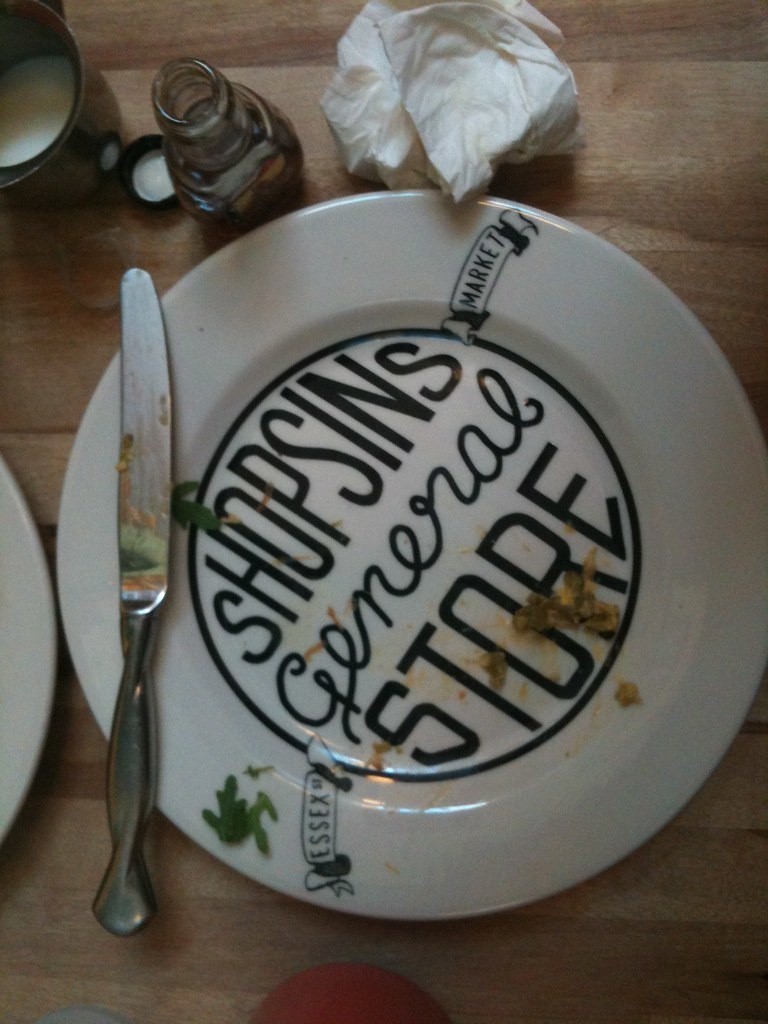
[93,268,171,937]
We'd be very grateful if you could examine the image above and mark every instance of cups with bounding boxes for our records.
[0,0,121,211]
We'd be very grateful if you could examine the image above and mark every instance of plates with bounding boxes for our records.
[55,190,768,922]
[0,451,60,852]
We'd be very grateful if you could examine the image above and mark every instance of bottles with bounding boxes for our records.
[152,56,305,239]
